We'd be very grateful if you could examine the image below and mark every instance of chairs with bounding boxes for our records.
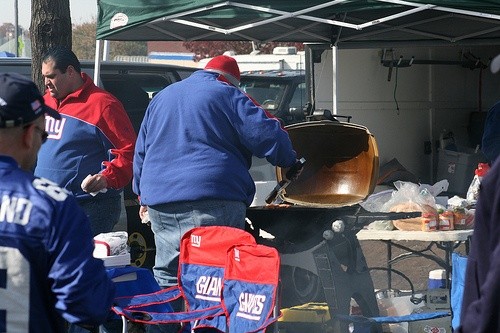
[102,225,284,333]
[341,252,468,333]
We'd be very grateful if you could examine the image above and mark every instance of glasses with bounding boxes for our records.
[24,124,48,143]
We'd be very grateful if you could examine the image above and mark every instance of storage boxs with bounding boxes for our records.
[438,146,481,195]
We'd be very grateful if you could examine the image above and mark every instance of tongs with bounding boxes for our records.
[264,157,306,203]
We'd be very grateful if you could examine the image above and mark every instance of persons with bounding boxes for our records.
[0,72,110,333]
[132,55,303,313]
[35,47,137,237]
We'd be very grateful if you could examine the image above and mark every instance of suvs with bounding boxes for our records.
[0,55,202,139]
[240,69,309,126]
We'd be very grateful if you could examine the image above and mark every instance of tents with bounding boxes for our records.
[94,0,500,119]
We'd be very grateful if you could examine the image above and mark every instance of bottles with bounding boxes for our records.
[423,211,466,232]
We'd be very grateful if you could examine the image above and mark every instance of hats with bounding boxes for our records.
[0,72,61,129]
[205,55,240,87]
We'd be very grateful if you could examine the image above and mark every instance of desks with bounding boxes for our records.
[356,228,473,310]
